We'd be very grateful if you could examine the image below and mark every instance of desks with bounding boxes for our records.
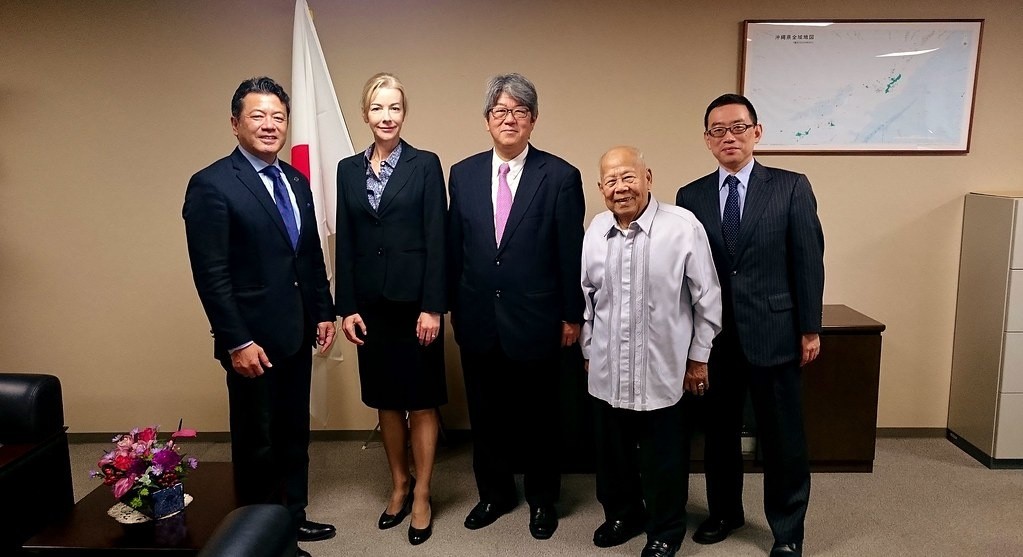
[22,462,237,557]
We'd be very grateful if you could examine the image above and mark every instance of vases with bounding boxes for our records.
[119,481,185,520]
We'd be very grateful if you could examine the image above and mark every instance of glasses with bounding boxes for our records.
[489,106,531,118]
[706,123,755,138]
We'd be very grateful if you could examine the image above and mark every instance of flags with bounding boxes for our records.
[289,0,355,281]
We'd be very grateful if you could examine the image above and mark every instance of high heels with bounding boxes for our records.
[379,475,416,530]
[408,496,432,546]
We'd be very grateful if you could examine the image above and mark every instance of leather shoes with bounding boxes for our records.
[527,496,558,539]
[643,527,686,557]
[594,515,645,547]
[695,511,745,544]
[295,520,335,541]
[465,494,519,529]
[770,538,803,557]
[296,547,311,557]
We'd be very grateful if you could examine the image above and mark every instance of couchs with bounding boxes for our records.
[199,505,300,557]
[0,373,74,557]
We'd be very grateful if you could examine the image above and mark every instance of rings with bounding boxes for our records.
[432,335,435,338]
[698,383,704,389]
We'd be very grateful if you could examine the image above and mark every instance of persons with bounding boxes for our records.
[578,146,721,557]
[335,72,449,546]
[182,77,338,557]
[677,93,824,557]
[449,72,585,540]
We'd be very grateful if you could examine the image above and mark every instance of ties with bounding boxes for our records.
[496,164,512,248]
[723,174,740,261]
[261,165,299,251]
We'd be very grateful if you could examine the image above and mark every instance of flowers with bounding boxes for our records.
[88,418,198,509]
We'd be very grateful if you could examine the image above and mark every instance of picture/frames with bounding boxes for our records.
[739,18,984,156]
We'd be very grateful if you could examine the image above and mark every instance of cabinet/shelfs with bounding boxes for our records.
[520,304,886,474]
[946,192,1023,469]
